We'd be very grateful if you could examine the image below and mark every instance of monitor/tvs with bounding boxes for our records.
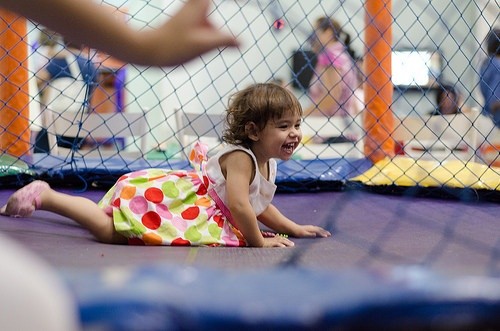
[391,49,443,90]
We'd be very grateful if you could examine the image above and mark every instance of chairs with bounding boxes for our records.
[44,106,148,160]
[297,115,364,160]
[393,107,479,162]
[174,107,231,162]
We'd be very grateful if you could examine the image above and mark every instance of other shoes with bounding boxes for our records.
[0,180,48,218]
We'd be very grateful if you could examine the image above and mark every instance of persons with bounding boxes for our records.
[0,0,241,67]
[302,17,362,116]
[0,83,331,248]
[35,37,99,157]
[479,26,500,130]
